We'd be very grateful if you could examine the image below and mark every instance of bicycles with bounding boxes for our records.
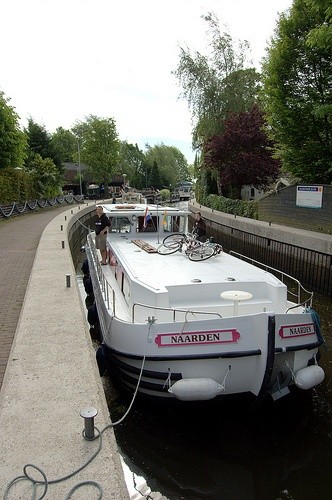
[157,222,222,262]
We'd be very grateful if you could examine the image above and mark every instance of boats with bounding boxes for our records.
[80,202,327,419]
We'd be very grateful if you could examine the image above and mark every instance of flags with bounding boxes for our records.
[144,207,150,229]
[162,211,167,225]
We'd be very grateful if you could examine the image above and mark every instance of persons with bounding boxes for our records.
[193,212,206,242]
[94,206,110,267]
[100,183,125,205]
